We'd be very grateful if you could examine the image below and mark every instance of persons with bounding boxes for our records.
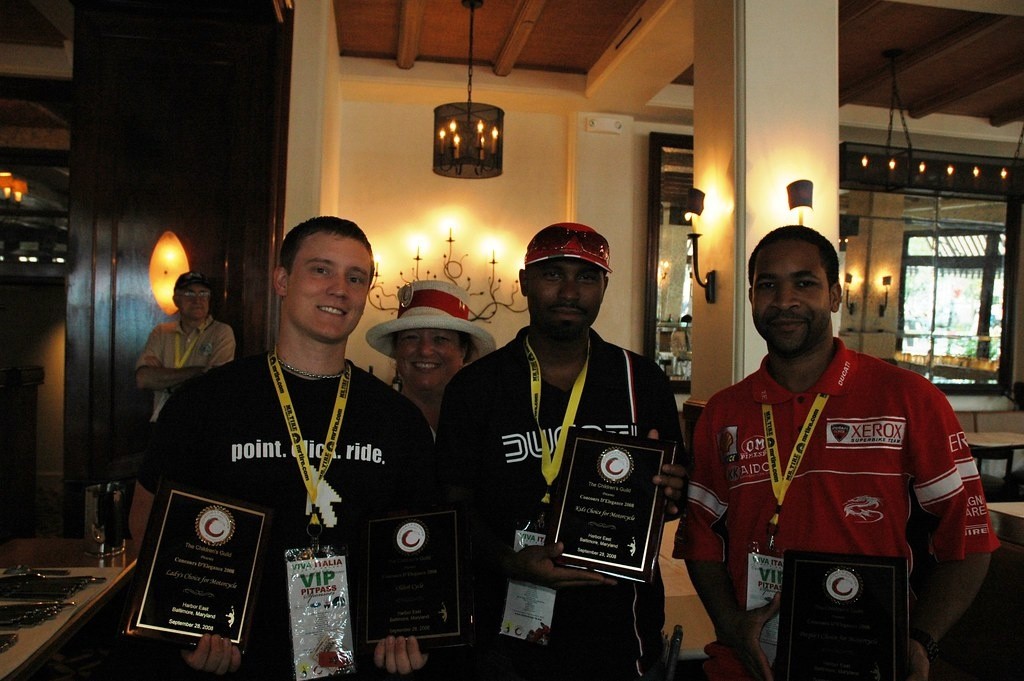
[437,221,692,681]
[151,216,441,681]
[682,225,995,681]
[365,279,496,441]
[135,273,237,430]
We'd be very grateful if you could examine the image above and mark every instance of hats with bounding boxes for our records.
[365,280,496,360]
[175,270,210,289]
[524,221,613,271]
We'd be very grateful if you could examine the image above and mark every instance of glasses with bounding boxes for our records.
[525,227,610,267]
[175,291,210,298]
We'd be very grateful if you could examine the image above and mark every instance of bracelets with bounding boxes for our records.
[910,627,940,669]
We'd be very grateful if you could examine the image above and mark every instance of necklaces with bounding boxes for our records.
[274,343,349,379]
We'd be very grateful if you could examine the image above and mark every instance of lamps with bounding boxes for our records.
[368,230,529,320]
[878,273,895,317]
[843,271,857,316]
[148,229,191,317]
[784,176,821,235]
[432,0,507,181]
[841,139,1024,202]
[678,187,719,306]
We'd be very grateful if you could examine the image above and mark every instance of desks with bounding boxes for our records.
[964,431,1024,479]
[985,502,1024,518]
